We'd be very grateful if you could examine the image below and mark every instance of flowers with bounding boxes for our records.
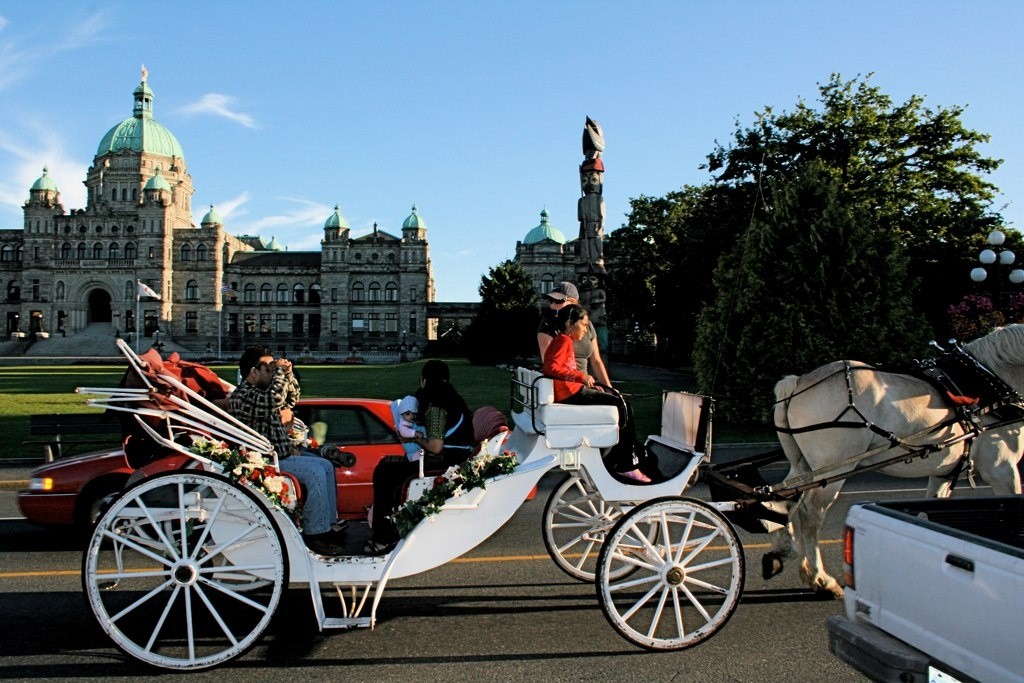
[189,437,309,528]
[385,438,520,538]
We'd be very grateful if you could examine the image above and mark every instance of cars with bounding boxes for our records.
[13,391,538,552]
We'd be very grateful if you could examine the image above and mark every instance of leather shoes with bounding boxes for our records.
[306,538,343,555]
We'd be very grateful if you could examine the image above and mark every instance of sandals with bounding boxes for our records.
[355,540,398,554]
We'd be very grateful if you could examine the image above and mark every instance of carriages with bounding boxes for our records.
[68,320,1024,674]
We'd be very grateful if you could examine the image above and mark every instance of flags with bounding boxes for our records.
[139,283,162,301]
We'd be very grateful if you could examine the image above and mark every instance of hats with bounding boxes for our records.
[543,281,580,299]
[399,395,419,414]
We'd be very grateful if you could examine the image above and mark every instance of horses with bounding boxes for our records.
[761,323,1024,601]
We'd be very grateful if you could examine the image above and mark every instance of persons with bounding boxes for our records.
[537,281,655,483]
[398,393,426,462]
[364,360,475,555]
[226,347,357,556]
[245,344,316,363]
[399,341,419,356]
[204,343,215,356]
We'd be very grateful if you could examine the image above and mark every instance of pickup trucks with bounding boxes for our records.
[822,493,1024,683]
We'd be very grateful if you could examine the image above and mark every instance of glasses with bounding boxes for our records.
[549,297,572,304]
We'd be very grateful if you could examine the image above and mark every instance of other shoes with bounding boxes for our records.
[617,469,652,482]
[330,519,349,532]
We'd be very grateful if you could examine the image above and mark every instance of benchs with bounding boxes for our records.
[399,406,509,510]
[510,361,628,448]
[22,413,122,459]
